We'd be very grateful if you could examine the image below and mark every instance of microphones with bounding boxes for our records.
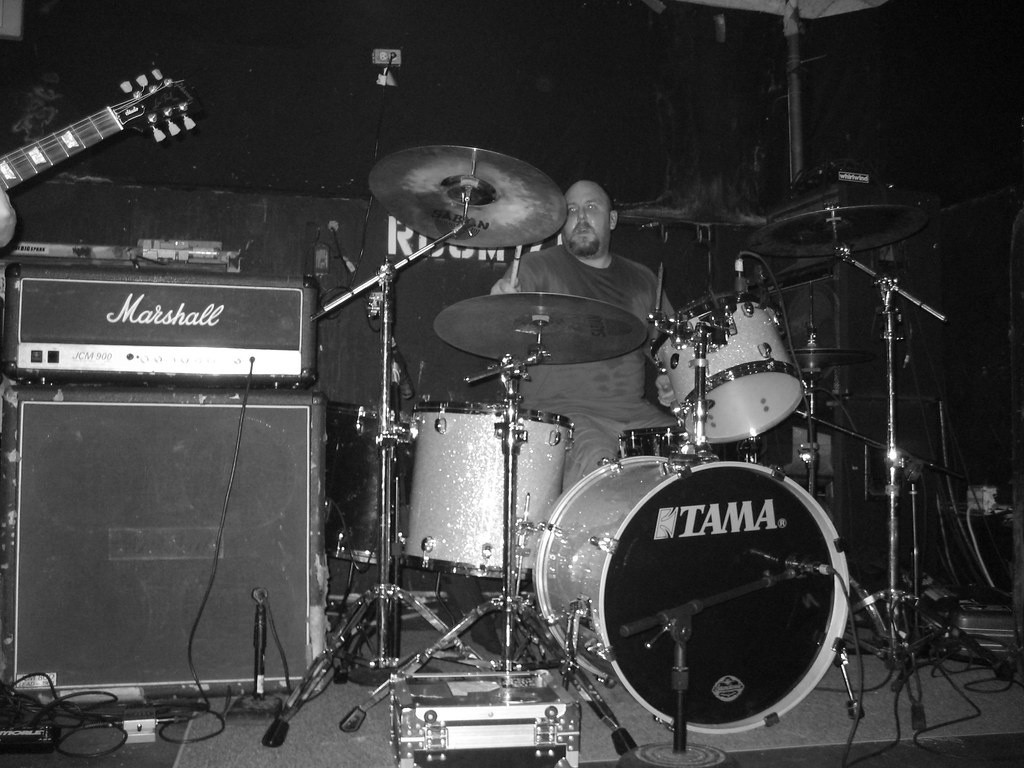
[391,336,414,399]
[749,548,835,575]
[734,254,748,297]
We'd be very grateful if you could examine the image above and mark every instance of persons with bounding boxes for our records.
[490,176,680,496]
[0,0,23,250]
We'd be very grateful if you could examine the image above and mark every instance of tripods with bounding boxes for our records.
[260,241,638,757]
[812,225,1014,731]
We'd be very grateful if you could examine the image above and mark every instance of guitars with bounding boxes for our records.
[1,66,199,192]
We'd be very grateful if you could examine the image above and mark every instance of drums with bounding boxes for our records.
[400,399,574,583]
[323,400,414,567]
[530,455,851,735]
[651,294,803,444]
[619,423,687,459]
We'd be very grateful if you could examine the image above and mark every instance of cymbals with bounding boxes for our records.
[434,292,648,366]
[788,344,875,367]
[743,203,930,258]
[369,145,569,249]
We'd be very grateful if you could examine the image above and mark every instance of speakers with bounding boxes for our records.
[0,386,330,706]
